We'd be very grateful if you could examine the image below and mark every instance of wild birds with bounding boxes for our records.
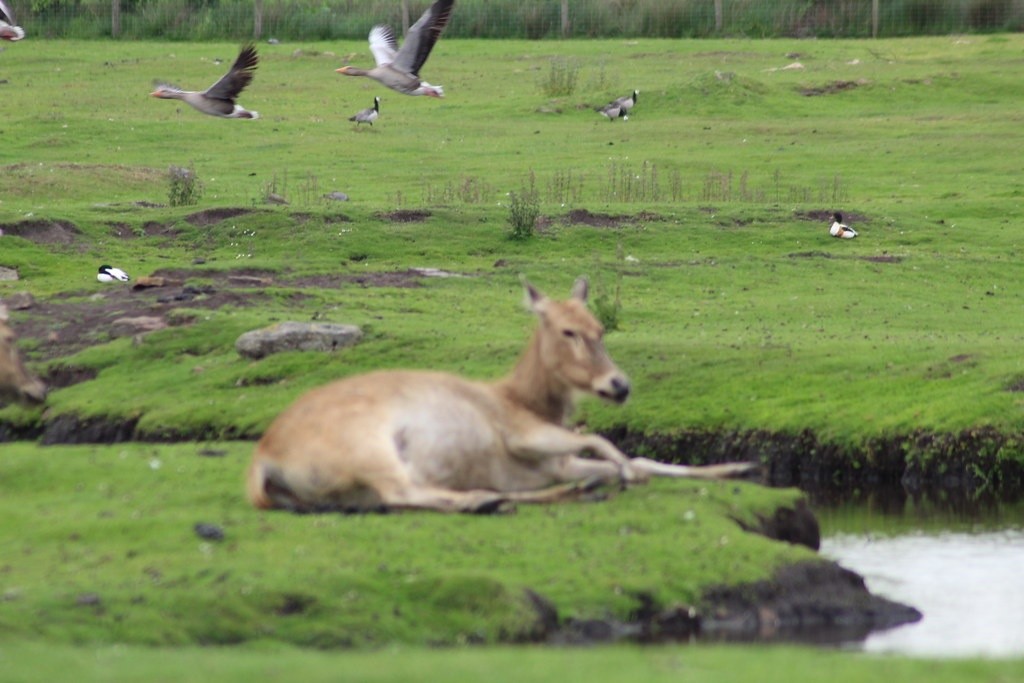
[829,212,859,240]
[97,264,130,284]
[0,0,26,44]
[333,0,455,99]
[146,41,258,121]
[347,97,380,128]
[593,89,641,124]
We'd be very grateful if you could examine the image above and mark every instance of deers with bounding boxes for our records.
[244,277,758,515]
